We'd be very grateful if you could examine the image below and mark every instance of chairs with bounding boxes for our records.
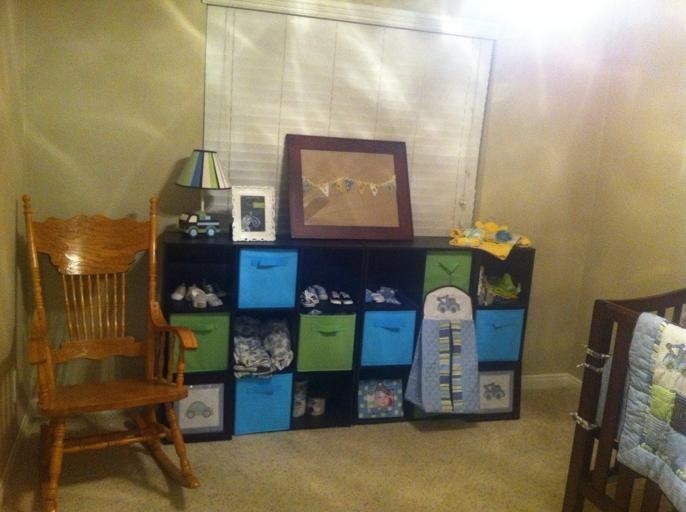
[16,194,202,512]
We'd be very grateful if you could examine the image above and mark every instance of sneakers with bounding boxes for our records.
[170,280,223,309]
[301,284,354,307]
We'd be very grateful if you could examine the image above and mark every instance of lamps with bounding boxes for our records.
[175,146,229,236]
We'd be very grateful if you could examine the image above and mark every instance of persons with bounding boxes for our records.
[373,385,394,411]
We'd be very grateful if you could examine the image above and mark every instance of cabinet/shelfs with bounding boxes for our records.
[159,222,535,443]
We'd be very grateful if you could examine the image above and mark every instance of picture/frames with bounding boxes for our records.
[228,133,417,244]
